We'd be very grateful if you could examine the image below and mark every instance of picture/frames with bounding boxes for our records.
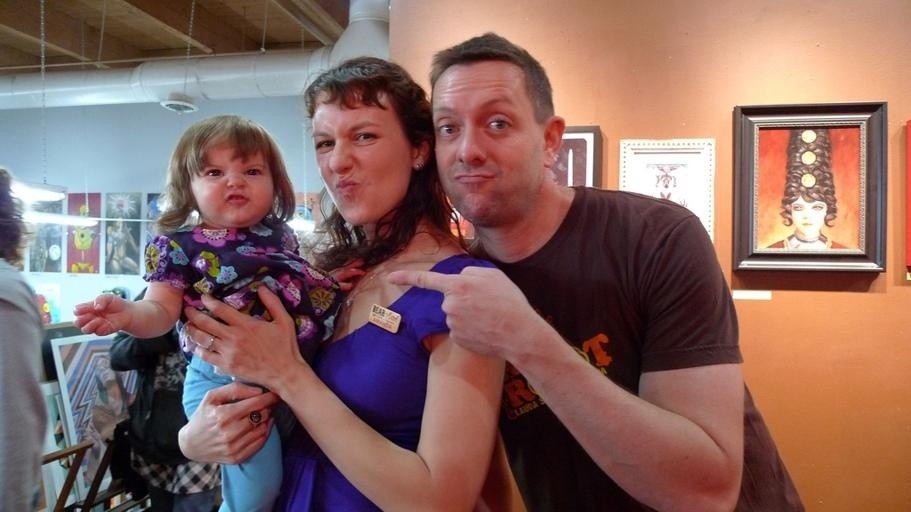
[619,137,717,246]
[447,123,602,246]
[730,101,887,273]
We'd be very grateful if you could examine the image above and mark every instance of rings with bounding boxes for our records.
[249,411,263,429]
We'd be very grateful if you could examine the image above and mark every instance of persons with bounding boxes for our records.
[108,284,221,512]
[186,55,507,511]
[72,115,368,512]
[106,216,139,274]
[766,128,849,249]
[0,166,49,511]
[384,32,805,511]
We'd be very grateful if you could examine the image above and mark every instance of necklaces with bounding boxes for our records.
[343,274,375,308]
